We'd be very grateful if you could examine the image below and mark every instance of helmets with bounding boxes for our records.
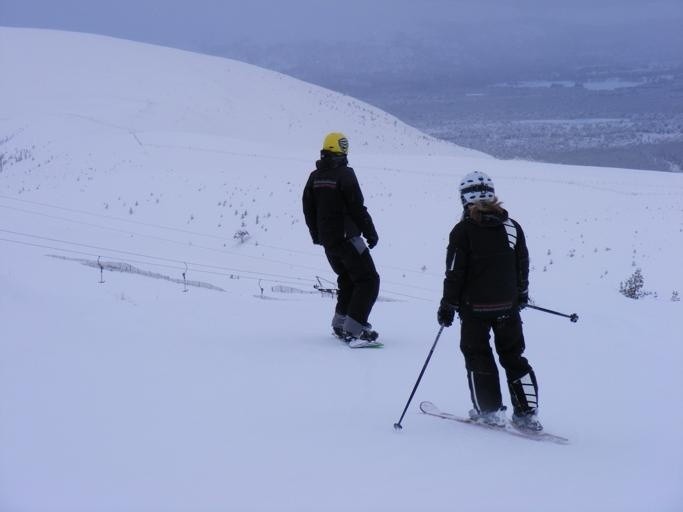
[460,170,494,206]
[323,132,348,153]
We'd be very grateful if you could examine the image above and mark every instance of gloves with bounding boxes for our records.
[519,297,528,312]
[367,239,377,249]
[437,305,454,327]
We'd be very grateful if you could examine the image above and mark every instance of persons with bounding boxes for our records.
[435,171,544,434]
[300,131,381,341]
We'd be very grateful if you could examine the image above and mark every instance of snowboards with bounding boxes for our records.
[348,336,383,348]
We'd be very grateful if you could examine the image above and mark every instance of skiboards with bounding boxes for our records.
[419,399,570,444]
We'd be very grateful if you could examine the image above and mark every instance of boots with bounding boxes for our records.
[332,313,378,340]
[512,412,543,432]
[469,408,507,426]
